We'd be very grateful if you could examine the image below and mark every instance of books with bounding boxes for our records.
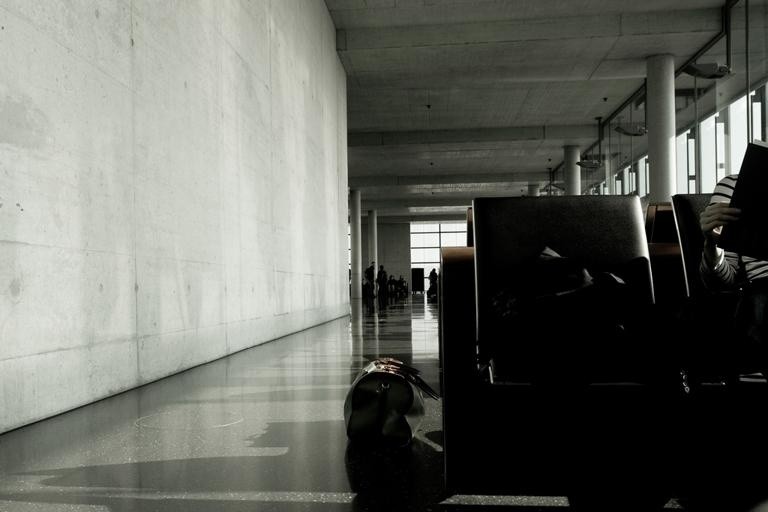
[716,139,768,264]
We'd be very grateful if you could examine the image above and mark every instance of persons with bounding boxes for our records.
[362,261,408,317]
[428,267,438,298]
[699,173,767,382]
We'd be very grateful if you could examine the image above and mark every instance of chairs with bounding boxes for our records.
[439,194,768,512]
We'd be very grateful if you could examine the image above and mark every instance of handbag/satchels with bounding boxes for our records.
[344,357,440,445]
